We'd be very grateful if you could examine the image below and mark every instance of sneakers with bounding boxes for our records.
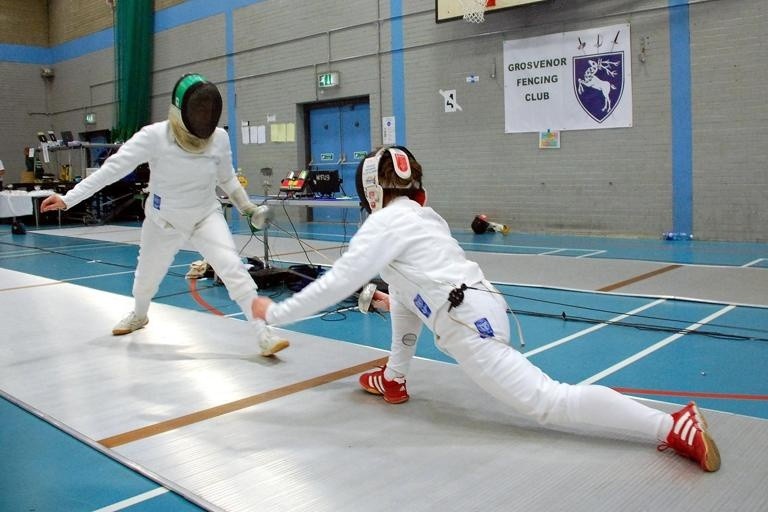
[358,364,409,404]
[111,310,150,335]
[257,329,290,357]
[666,399,721,473]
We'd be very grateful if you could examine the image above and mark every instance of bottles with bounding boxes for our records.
[660,231,694,241]
[236,168,248,194]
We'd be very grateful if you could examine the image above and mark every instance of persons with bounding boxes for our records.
[39,72,290,359]
[250,143,722,475]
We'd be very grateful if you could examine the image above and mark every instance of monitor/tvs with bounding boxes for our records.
[279,168,309,199]
[309,169,343,199]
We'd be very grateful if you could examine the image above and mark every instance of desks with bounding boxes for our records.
[219,194,364,270]
[0,189,62,229]
